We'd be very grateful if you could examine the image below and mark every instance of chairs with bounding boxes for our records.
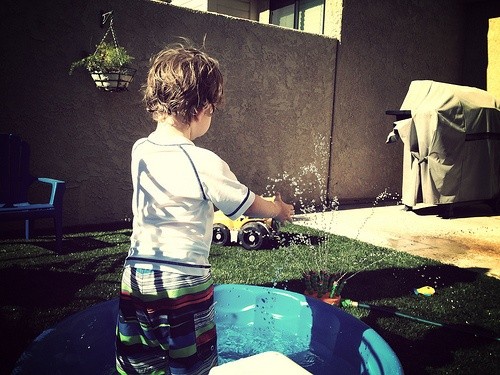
[0,134,65,256]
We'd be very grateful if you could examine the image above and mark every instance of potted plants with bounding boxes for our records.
[69,43,137,92]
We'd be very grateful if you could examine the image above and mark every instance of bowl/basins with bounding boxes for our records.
[7,285,403,375]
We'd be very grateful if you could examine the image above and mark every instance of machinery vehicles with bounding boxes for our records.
[212,194,279,249]
